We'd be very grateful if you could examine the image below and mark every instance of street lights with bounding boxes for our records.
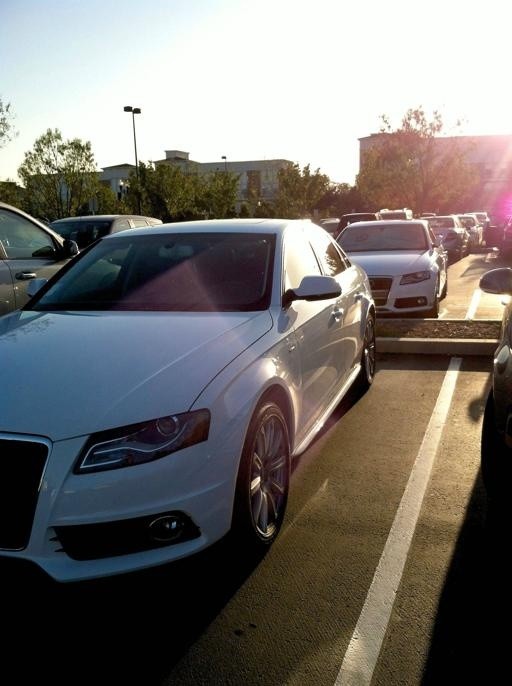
[222,156,226,172]
[124,106,141,174]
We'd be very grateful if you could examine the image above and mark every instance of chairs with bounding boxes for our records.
[111,263,271,308]
